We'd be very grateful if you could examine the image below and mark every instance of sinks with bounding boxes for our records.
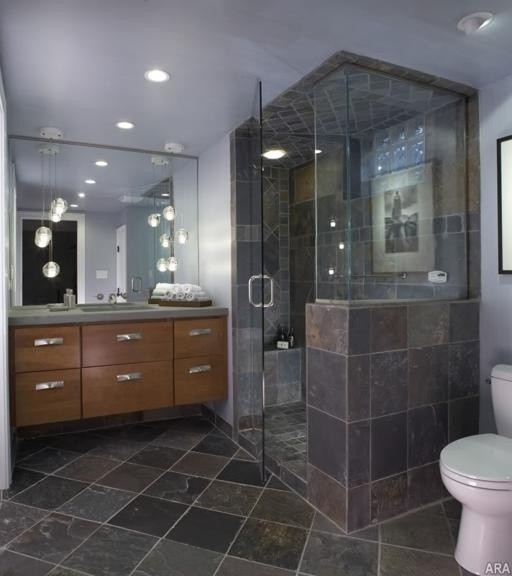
[80,305,119,312]
[115,305,162,311]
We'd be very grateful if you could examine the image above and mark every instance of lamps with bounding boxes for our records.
[148,156,177,273]
[163,142,190,272]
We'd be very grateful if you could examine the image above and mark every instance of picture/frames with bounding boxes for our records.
[368,156,438,275]
[496,134,512,275]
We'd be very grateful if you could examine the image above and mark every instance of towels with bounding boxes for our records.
[164,284,208,302]
[151,282,172,300]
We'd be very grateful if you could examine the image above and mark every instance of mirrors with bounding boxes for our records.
[8,135,199,309]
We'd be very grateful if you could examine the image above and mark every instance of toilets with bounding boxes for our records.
[438,363,512,576]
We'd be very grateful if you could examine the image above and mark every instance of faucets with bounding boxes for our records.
[108,293,116,304]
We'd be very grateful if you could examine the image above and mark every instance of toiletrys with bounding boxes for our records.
[66,289,76,310]
[63,289,69,306]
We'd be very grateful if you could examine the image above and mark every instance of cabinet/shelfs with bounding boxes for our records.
[9,315,227,427]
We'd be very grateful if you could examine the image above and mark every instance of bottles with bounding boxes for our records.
[274,326,297,350]
